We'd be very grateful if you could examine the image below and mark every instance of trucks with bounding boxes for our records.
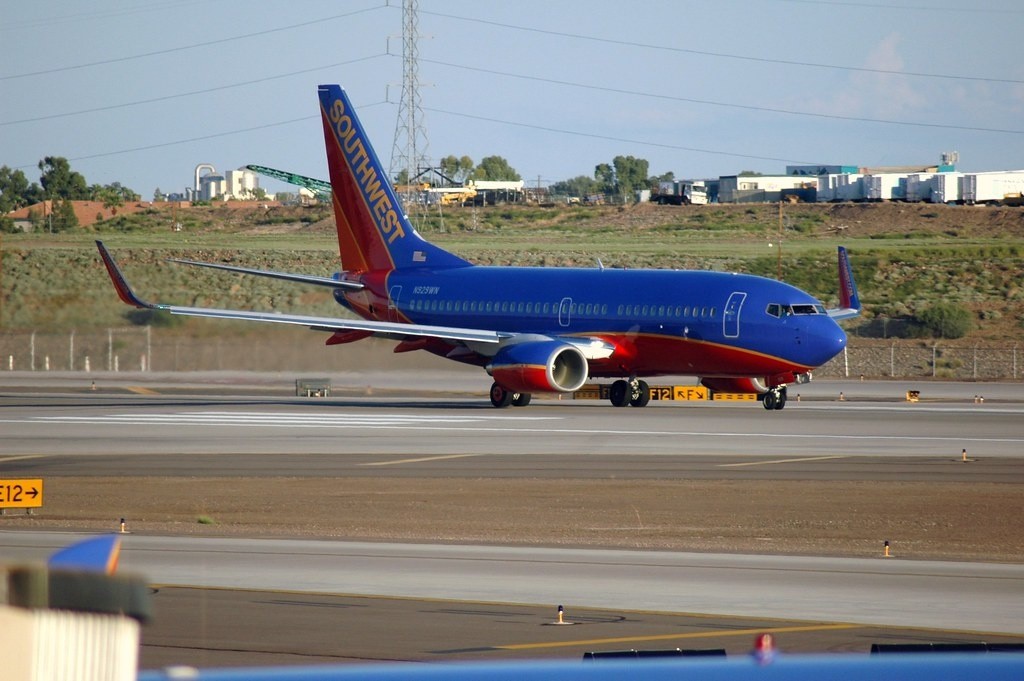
[651,184,709,206]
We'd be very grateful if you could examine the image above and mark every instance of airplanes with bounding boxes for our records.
[96,82,865,414]
